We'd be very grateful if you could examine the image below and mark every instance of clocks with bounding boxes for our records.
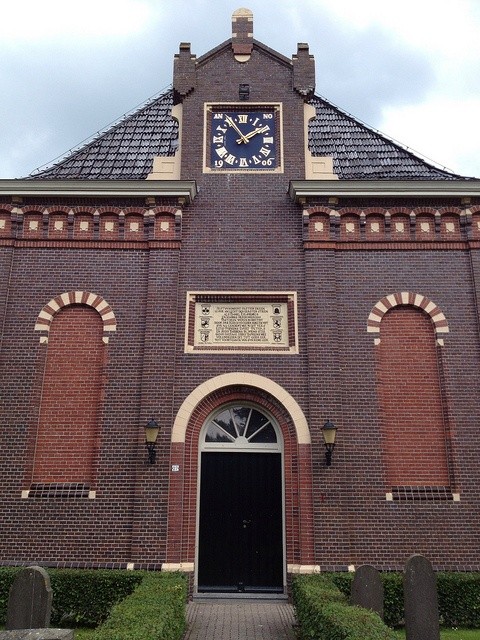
[204,102,284,175]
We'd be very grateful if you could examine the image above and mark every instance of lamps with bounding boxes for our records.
[143,418,161,464]
[320,420,338,467]
